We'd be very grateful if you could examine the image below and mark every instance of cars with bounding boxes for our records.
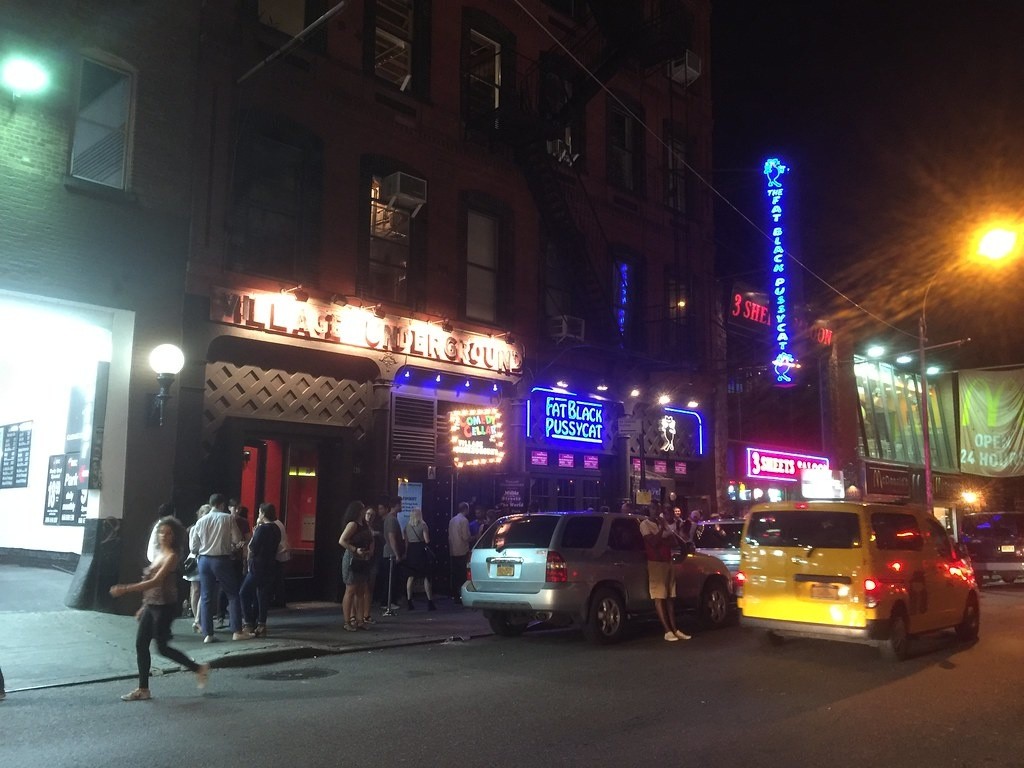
[962,513,1024,588]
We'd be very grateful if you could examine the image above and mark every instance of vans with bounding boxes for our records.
[735,500,980,662]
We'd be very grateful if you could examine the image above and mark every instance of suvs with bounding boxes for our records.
[690,517,783,575]
[459,510,736,647]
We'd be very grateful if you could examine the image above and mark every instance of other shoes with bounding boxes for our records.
[191,622,203,634]
[381,604,400,610]
[344,616,375,631]
[196,663,210,690]
[675,630,692,640]
[203,635,217,643]
[254,626,267,637]
[121,689,150,701]
[663,631,679,642]
[232,632,256,641]
[215,617,224,628]
[242,625,256,635]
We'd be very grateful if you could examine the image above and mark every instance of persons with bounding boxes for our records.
[660,493,700,539]
[182,494,290,643]
[448,502,497,604]
[640,500,692,641]
[109,515,208,699]
[337,495,437,633]
[146,503,177,562]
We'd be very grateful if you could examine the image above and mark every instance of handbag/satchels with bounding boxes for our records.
[350,547,368,574]
[424,545,439,566]
[182,558,197,575]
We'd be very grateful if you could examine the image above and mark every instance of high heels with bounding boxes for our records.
[428,601,436,611]
[408,599,415,610]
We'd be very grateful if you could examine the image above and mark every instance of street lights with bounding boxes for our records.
[918,226,1020,516]
